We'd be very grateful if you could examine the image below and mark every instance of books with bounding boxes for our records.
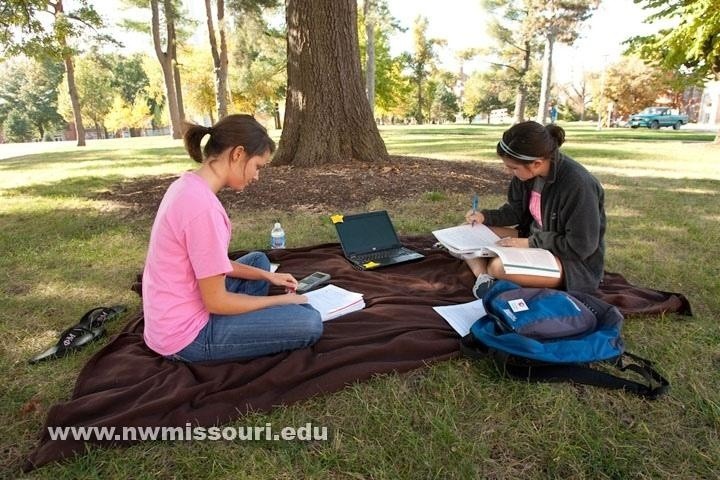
[431,220,503,254]
[449,244,561,280]
[299,282,366,323]
[432,297,488,339]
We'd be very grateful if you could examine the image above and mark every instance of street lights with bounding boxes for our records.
[597,53,612,130]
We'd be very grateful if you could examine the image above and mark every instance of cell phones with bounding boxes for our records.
[296,271,331,292]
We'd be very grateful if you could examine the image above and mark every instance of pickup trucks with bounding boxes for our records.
[628,107,689,130]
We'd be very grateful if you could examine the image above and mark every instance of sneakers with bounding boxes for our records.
[472,273,499,300]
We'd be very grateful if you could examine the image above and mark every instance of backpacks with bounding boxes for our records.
[459,277,669,400]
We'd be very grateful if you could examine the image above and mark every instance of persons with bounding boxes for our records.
[453,119,608,301]
[140,113,325,364]
[550,104,557,122]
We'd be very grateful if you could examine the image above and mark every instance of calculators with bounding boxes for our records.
[295,271,330,293]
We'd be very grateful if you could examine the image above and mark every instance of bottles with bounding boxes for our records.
[270,221,286,249]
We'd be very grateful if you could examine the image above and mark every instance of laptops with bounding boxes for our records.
[334,210,426,271]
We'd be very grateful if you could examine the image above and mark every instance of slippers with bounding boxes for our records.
[28,327,106,364]
[61,306,127,338]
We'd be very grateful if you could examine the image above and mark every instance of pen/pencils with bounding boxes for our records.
[471,192,479,228]
